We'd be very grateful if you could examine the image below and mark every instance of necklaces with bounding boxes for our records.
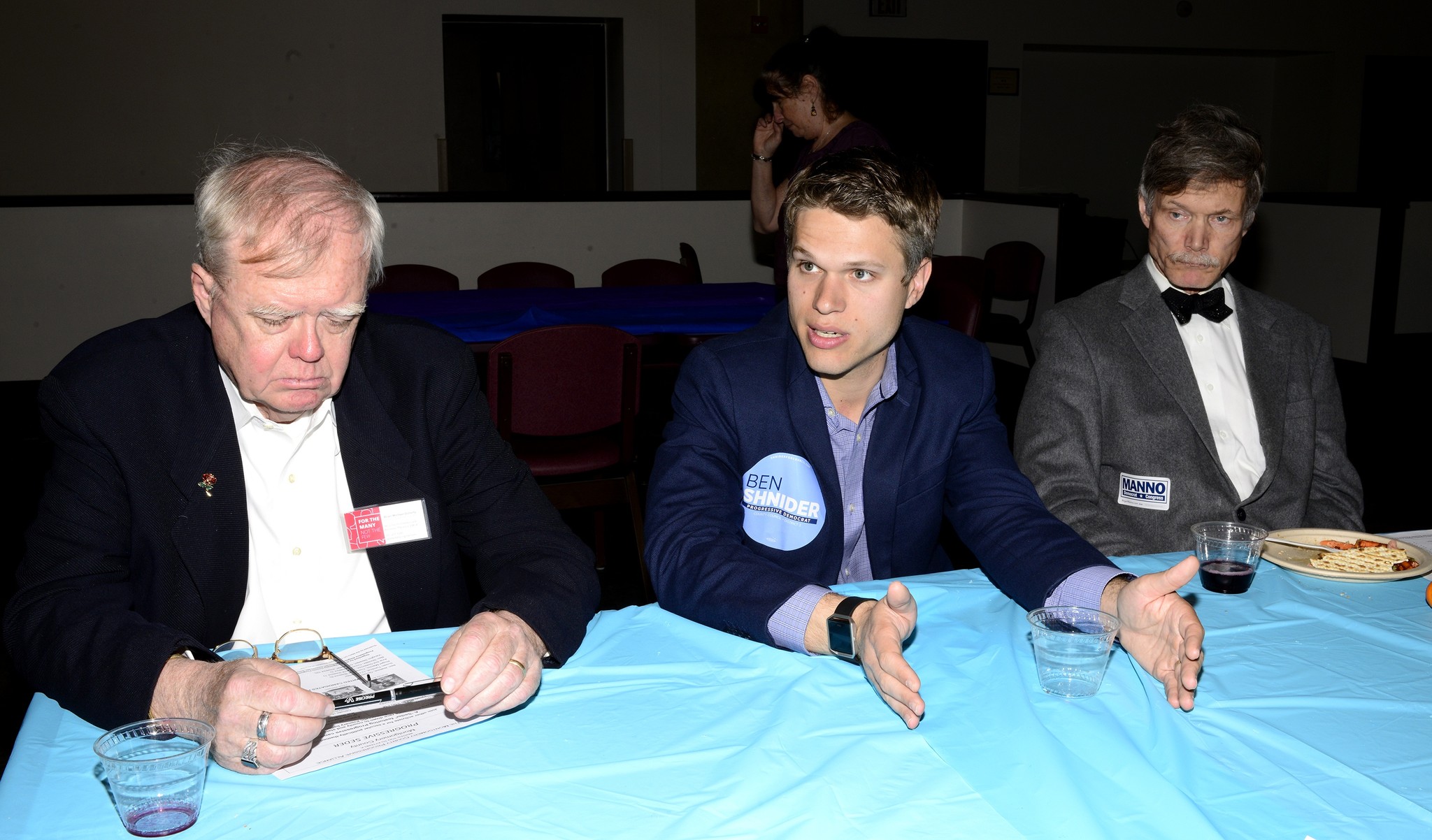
[808,127,831,154]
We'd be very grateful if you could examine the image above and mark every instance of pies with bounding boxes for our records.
[1309,546,1408,572]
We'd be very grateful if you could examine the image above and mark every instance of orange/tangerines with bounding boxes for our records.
[1426,580,1432,608]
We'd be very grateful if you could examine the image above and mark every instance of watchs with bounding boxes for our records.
[826,596,880,665]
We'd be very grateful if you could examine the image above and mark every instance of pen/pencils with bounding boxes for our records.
[333,681,443,708]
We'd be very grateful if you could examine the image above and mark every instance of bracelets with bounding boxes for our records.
[145,653,185,729]
[751,153,773,163]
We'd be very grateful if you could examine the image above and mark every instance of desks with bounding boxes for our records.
[0,530,1431,840]
[365,279,786,372]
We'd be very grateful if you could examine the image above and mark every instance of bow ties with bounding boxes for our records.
[1160,286,1234,325]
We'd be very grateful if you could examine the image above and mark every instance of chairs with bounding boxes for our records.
[368,240,1046,585]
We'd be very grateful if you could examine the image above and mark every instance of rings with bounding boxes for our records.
[257,711,272,741]
[240,738,260,768]
[509,659,527,679]
[1174,660,1180,669]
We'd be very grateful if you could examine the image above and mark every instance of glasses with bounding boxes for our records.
[210,628,373,689]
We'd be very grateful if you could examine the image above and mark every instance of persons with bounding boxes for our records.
[644,150,1207,730]
[0,141,601,775]
[750,24,894,289]
[1013,102,1365,558]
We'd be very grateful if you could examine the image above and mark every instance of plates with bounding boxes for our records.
[1258,528,1432,584]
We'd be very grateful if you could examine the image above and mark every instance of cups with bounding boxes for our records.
[1026,607,1122,699]
[93,716,215,838]
[1191,521,1268,594]
